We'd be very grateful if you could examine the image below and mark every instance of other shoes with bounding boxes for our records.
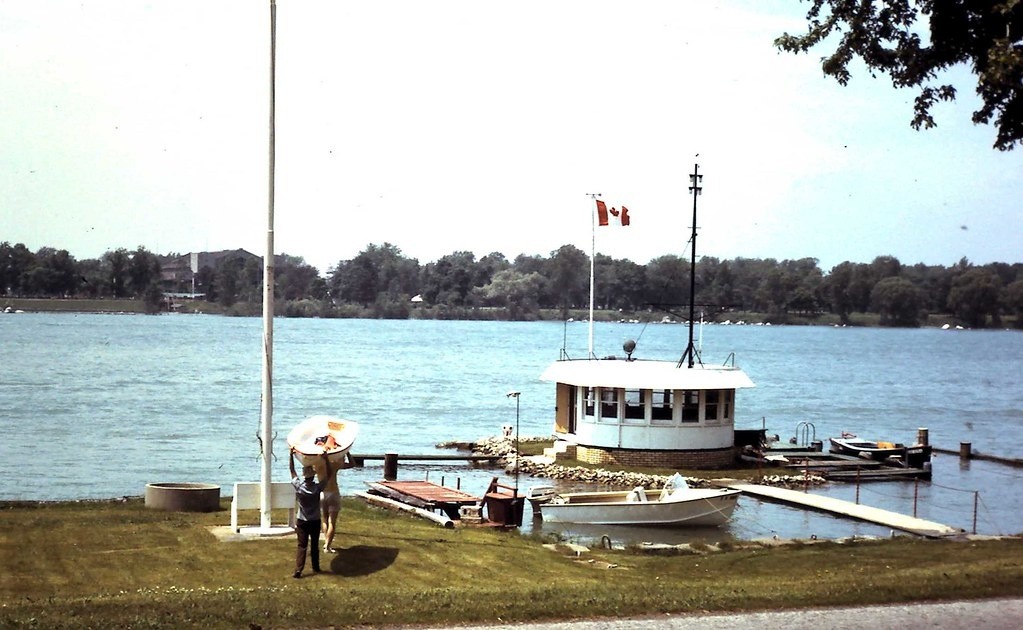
[312,570,321,575]
[323,544,336,554]
[292,572,302,578]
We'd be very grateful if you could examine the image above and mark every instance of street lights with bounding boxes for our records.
[675,164,705,370]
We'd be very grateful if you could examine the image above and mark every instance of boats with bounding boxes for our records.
[830,436,932,469]
[540,488,741,525]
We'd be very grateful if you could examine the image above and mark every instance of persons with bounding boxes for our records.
[314,452,356,553]
[289,446,332,578]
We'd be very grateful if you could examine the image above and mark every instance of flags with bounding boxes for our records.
[596,200,630,226]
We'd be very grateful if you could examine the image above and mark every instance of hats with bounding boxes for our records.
[301,466,317,478]
